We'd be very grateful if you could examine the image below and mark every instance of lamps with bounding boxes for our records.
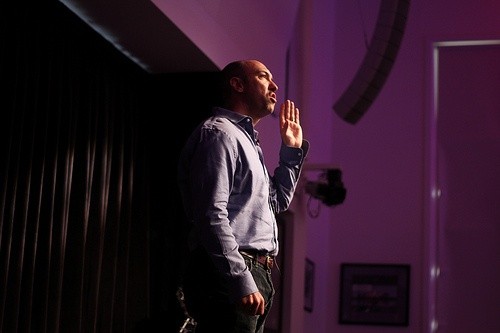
[304,168,348,218]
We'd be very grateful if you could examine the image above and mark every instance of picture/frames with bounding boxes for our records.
[337,262,411,327]
[302,258,315,313]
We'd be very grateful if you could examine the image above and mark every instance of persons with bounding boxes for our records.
[192,60,310,333]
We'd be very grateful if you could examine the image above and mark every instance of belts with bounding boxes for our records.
[216,242,275,270]
